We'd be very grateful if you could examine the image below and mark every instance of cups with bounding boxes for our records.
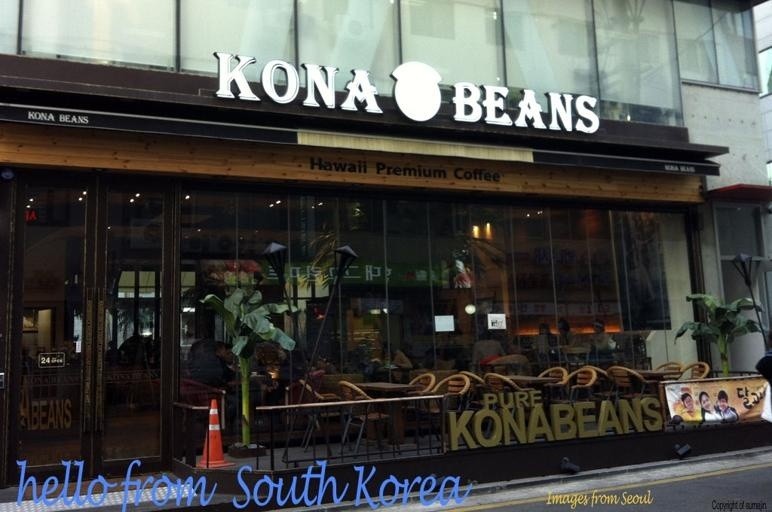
[252,372,257,375]
[388,364,395,367]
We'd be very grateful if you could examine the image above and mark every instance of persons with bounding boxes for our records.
[713,388,741,425]
[673,390,701,421]
[14,315,621,432]
[697,391,724,423]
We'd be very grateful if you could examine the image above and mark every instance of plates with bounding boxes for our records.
[386,367,398,369]
[251,375,257,376]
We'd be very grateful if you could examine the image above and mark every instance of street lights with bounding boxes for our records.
[281,242,359,462]
[732,253,771,357]
[262,240,334,456]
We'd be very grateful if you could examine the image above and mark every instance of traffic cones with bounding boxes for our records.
[196,399,238,469]
